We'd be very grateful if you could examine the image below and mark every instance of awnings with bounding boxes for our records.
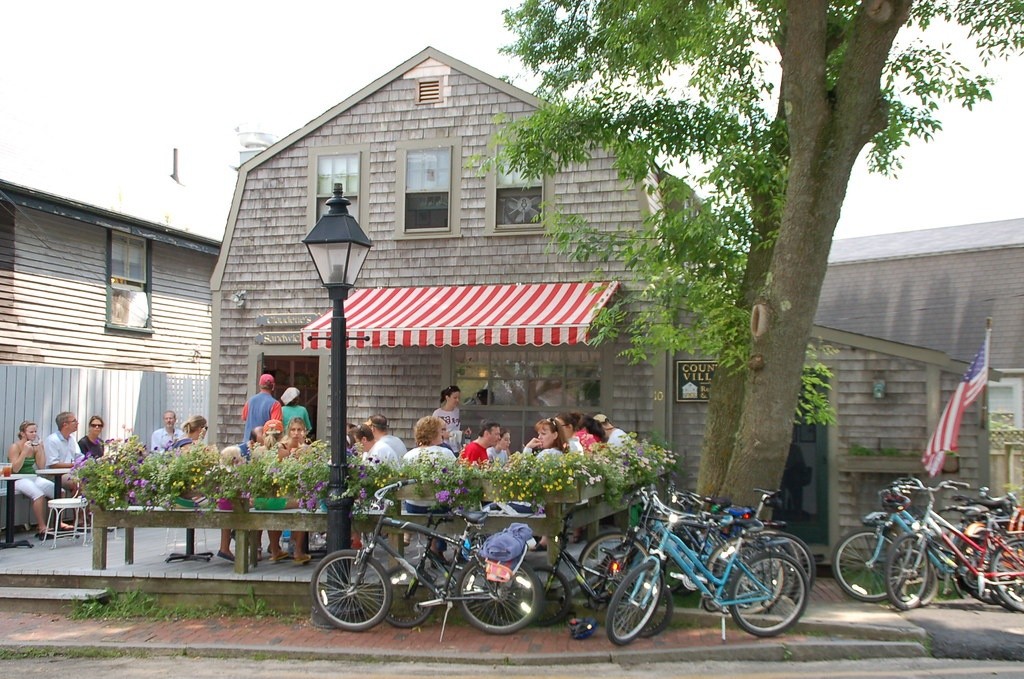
[299,280,620,351]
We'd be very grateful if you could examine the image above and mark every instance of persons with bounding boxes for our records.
[78,416,105,460]
[241,374,283,444]
[783,444,813,520]
[535,378,576,407]
[433,385,472,459]
[43,412,91,526]
[171,415,208,454]
[151,410,184,454]
[217,418,326,566]
[401,416,459,569]
[346,414,407,550]
[9,421,75,535]
[281,387,312,436]
[522,414,584,552]
[458,421,510,507]
[575,410,631,455]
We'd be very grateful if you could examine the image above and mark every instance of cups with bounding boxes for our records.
[2,463,12,477]
[0,463,5,477]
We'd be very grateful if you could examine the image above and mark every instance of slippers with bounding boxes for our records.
[268,551,290,561]
[39,527,54,540]
[59,524,74,531]
[292,552,312,565]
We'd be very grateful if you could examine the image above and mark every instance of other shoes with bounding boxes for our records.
[432,553,469,570]
[215,550,235,561]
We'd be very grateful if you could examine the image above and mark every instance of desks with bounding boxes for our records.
[0,474,36,549]
[35,469,73,540]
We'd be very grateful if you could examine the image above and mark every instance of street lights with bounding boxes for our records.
[301,183,375,630]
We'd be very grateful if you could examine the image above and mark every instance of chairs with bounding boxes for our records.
[162,528,207,562]
[89,508,122,542]
[38,481,88,549]
[161,526,213,563]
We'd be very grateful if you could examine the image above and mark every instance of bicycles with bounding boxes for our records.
[515,482,815,647]
[374,505,546,630]
[831,472,1024,612]
[310,479,544,643]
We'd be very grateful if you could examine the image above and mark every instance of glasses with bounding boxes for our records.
[25,433,40,447]
[202,427,208,430]
[90,424,104,427]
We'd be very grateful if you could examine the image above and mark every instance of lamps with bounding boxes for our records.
[232,291,247,311]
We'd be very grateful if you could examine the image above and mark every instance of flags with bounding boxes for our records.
[921,329,990,480]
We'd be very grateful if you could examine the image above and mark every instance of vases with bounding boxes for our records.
[832,456,958,473]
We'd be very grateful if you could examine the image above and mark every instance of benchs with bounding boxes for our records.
[1,487,23,498]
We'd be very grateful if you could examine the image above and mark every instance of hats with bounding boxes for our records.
[593,414,607,423]
[258,373,274,387]
[263,418,283,438]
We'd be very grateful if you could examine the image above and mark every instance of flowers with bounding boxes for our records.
[77,430,683,508]
[844,444,952,457]
